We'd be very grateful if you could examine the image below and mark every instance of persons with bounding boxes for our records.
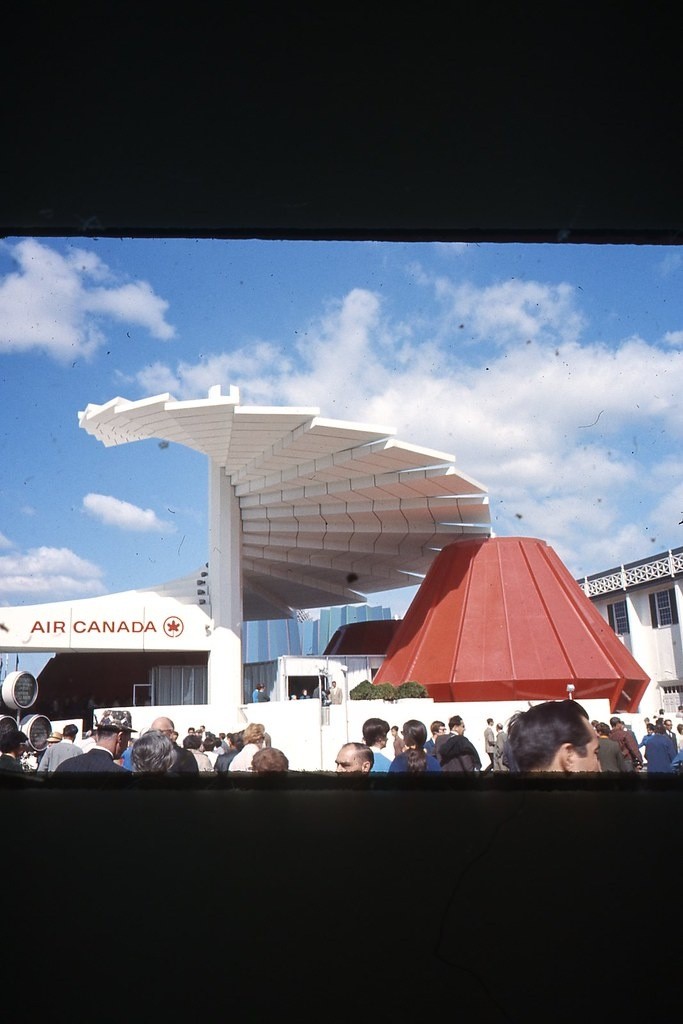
[0,709,289,772]
[252,683,270,702]
[637,706,683,773]
[484,699,643,772]
[335,714,482,773]
[313,681,342,706]
[291,689,311,699]
[52,695,151,709]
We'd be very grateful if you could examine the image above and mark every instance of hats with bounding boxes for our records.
[95,710,138,732]
[46,732,62,743]
